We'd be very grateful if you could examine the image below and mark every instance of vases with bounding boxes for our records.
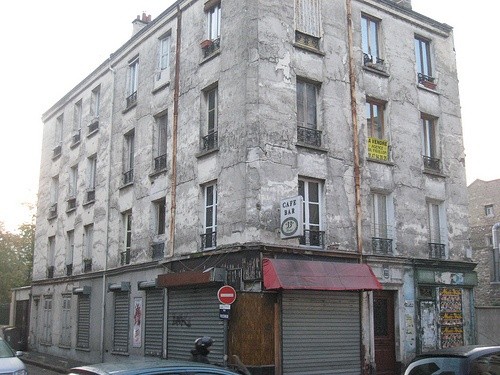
[421,79,437,89]
[200,39,213,50]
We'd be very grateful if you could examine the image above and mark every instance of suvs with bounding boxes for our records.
[62,358,243,375]
[403,345,500,375]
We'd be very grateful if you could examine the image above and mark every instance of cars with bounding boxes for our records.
[0,336,28,375]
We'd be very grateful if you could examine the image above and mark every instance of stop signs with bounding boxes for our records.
[217,285,236,304]
